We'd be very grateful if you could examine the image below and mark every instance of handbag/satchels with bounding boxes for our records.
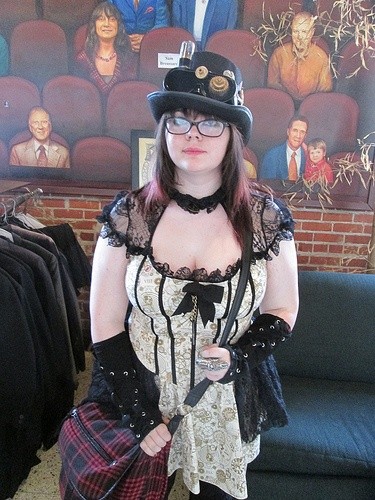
[57,398,173,500]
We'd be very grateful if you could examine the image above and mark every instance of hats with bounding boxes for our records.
[147,52,253,149]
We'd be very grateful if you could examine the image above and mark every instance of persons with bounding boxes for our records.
[88,52,300,500]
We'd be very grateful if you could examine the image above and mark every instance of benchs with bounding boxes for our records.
[245,270,375,500]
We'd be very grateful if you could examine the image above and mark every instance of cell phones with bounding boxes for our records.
[196,357,227,369]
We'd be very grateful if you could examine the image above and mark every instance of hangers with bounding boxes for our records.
[0,196,61,284]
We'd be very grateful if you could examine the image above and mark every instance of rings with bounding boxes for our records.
[196,359,229,371]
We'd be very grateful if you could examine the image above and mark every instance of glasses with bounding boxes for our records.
[165,116,231,137]
[162,67,237,103]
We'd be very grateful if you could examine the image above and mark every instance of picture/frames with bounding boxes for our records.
[0,0,375,211]
[131,129,157,192]
